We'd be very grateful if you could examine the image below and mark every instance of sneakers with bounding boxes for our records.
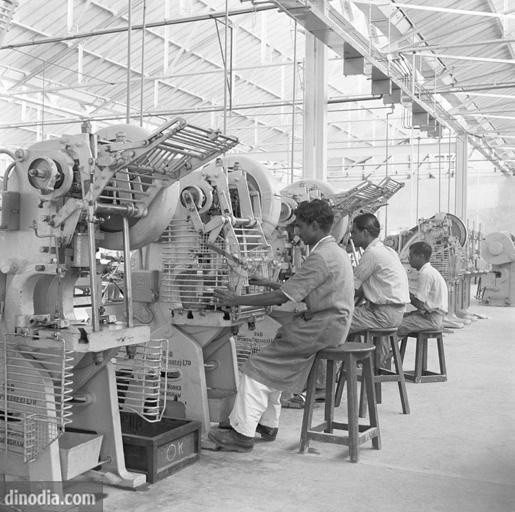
[208,430,254,451]
[256,423,278,441]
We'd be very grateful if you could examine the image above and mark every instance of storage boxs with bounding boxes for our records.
[119,411,202,484]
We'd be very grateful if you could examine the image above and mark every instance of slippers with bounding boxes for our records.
[291,393,325,404]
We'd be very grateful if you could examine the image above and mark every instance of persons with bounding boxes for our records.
[207,199,357,452]
[358,242,449,378]
[283,214,410,408]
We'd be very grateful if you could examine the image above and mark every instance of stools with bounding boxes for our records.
[300,329,447,464]
